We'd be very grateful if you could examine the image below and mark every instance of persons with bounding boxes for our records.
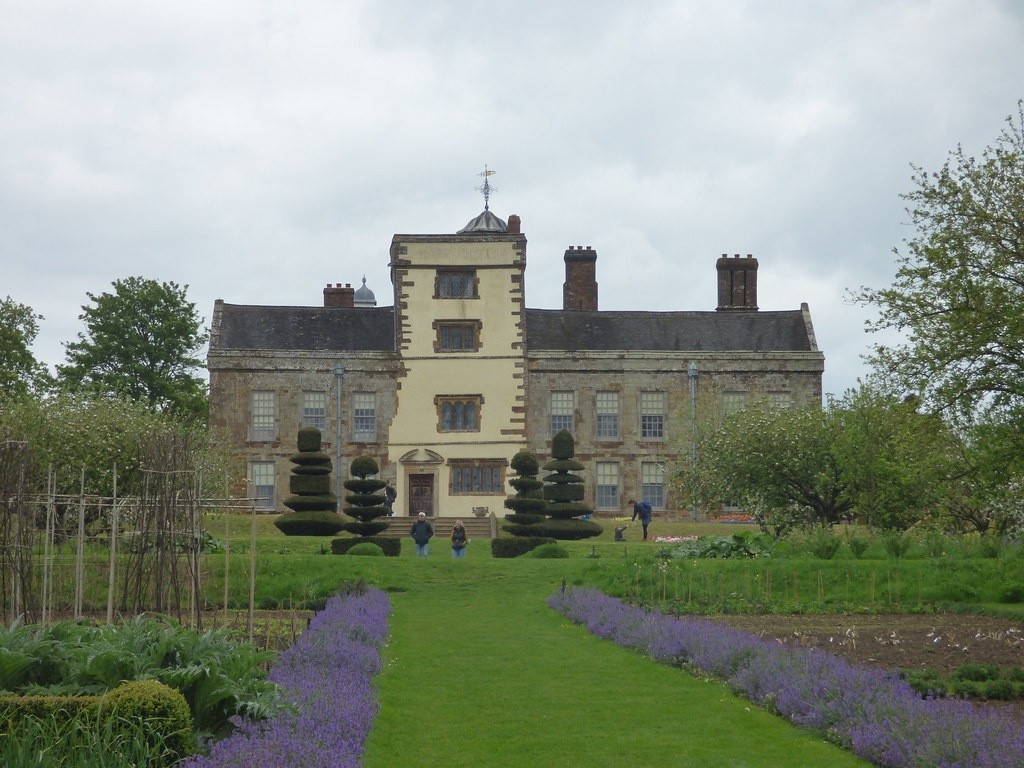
[615,525,628,541]
[410,512,434,557]
[450,520,468,558]
[628,499,650,539]
[384,480,397,516]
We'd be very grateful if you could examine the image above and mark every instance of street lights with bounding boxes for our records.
[688,361,699,521]
[334,357,344,514]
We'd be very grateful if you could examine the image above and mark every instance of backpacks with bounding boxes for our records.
[637,501,652,513]
